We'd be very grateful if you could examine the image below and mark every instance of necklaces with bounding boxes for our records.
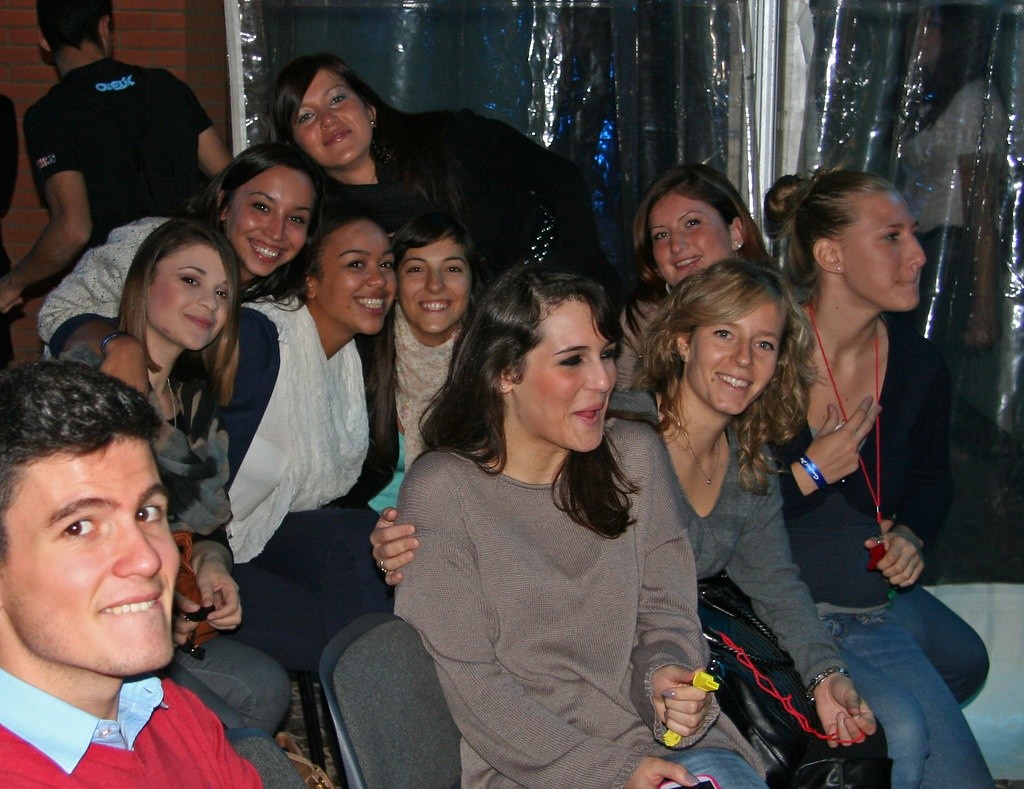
[678,422,720,484]
[168,377,177,428]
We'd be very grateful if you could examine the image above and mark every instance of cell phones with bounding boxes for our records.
[658,774,722,789]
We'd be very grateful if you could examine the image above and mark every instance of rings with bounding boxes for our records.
[380,560,389,573]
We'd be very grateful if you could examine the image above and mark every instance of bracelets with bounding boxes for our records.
[101,331,128,356]
[799,455,827,489]
[804,667,849,698]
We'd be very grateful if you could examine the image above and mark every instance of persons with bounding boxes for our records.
[370,259,895,789]
[763,171,995,789]
[392,268,774,789]
[634,163,990,699]
[898,0,1006,349]
[0,0,599,736]
[0,360,263,789]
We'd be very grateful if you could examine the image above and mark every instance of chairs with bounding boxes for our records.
[225,613,462,789]
